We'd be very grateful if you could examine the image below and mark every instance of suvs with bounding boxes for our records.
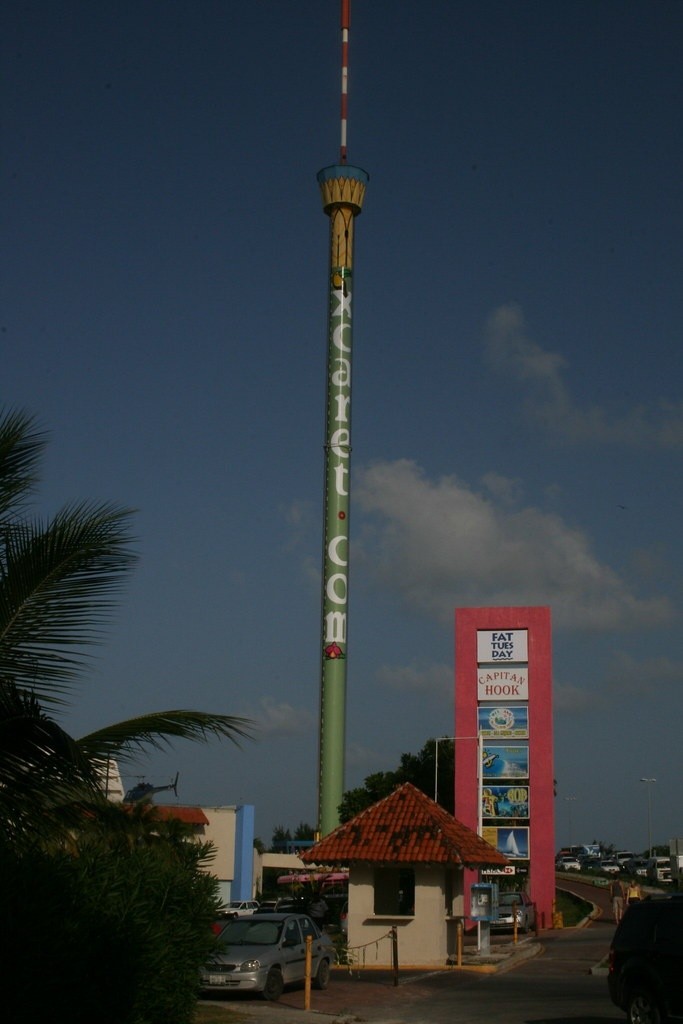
[607,893,683,1024]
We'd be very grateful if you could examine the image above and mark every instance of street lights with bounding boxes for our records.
[563,797,576,844]
[640,778,656,858]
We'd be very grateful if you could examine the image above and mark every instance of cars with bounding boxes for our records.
[197,913,335,1000]
[260,900,277,913]
[627,856,648,877]
[582,859,599,871]
[612,851,633,869]
[490,892,536,933]
[596,860,621,873]
[216,901,260,918]
[558,857,582,872]
[340,901,348,935]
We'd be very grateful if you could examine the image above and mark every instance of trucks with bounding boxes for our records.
[670,854,683,887]
[649,857,673,883]
[560,844,600,858]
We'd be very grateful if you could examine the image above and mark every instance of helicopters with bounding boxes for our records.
[119,770,179,803]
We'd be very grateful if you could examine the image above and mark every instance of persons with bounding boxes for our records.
[626,879,640,906]
[309,896,328,932]
[609,876,625,926]
